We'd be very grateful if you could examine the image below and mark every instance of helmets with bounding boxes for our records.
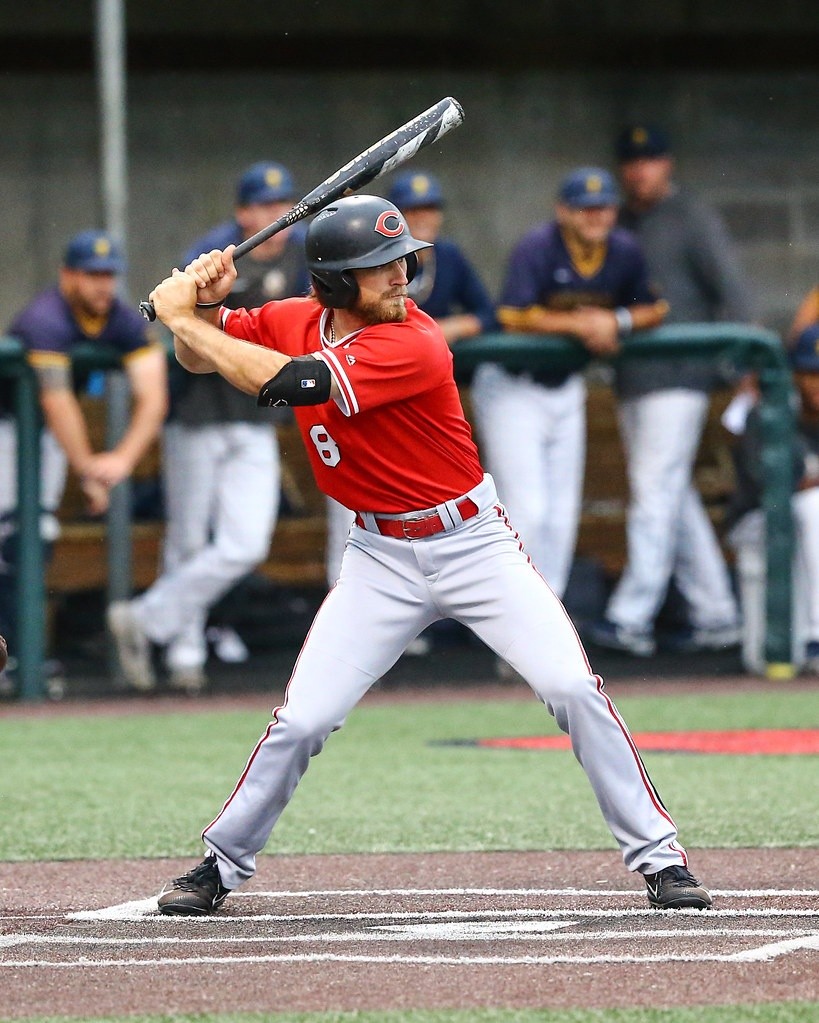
[615,124,673,160]
[391,168,442,208]
[306,194,435,308]
[792,322,819,374]
[238,160,295,205]
[65,228,122,273]
[559,167,624,209]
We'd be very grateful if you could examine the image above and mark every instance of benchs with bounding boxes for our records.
[42,382,751,678]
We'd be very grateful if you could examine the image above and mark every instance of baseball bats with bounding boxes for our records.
[138,94,467,322]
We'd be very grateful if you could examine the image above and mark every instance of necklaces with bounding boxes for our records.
[330,312,335,344]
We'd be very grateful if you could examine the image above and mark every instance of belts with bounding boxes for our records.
[355,497,479,539]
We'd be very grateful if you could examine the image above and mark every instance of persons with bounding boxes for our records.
[327,168,500,653]
[469,166,670,686]
[583,111,763,654]
[724,322,819,678]
[0,229,169,698]
[147,194,715,915]
[108,160,311,687]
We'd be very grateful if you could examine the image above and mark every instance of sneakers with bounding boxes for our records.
[643,865,713,910]
[165,665,208,692]
[158,856,232,914]
[0,657,66,704]
[803,656,819,679]
[106,601,155,690]
[368,619,746,693]
